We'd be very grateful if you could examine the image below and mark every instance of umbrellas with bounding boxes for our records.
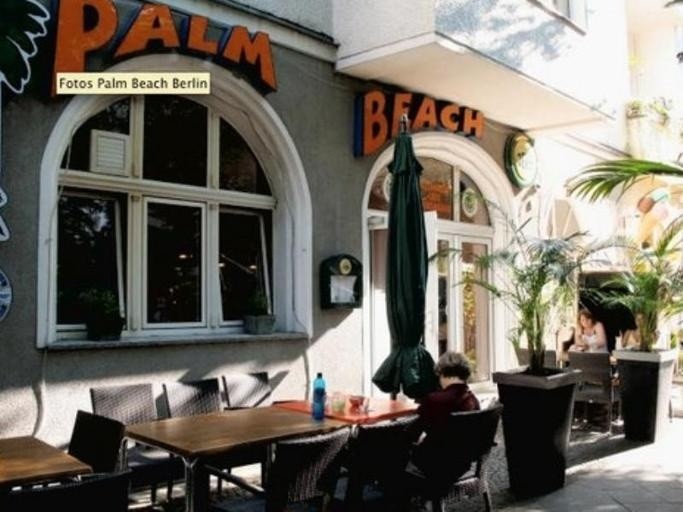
[369,114,441,402]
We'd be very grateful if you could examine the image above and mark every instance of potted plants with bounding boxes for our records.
[426,188,657,497]
[578,239,683,443]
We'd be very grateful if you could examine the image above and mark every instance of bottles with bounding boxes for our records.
[312,372,326,422]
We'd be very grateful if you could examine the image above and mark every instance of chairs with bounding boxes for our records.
[515,346,559,368]
[568,350,622,430]
[88,385,174,504]
[40,409,125,489]
[199,427,350,509]
[161,379,265,492]
[409,405,502,511]
[335,413,420,510]
[218,371,297,458]
[12,468,130,512]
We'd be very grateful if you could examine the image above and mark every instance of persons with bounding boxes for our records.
[615,312,660,363]
[571,307,610,358]
[364,349,481,498]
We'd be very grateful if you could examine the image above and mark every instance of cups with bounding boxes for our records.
[348,395,370,414]
[331,392,346,413]
